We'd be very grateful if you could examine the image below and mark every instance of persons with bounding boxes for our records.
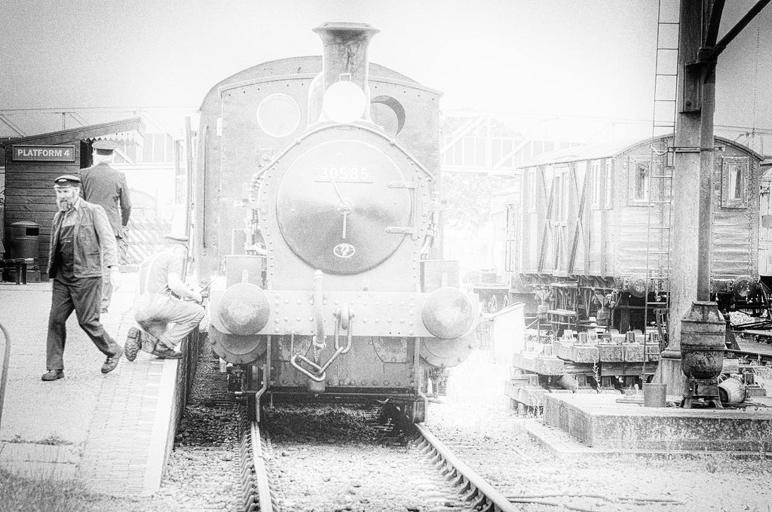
[78,140,133,315]
[123,234,206,363]
[40,174,124,382]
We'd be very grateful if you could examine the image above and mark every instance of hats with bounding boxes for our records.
[92,140,118,154]
[54,175,81,184]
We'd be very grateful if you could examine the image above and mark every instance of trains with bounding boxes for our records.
[167,13,483,425]
[487,137,763,379]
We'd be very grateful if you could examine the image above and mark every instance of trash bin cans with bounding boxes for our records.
[8,221,42,283]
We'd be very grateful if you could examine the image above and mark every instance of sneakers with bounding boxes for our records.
[152,340,184,359]
[42,369,64,381]
[124,327,142,361]
[101,344,124,373]
